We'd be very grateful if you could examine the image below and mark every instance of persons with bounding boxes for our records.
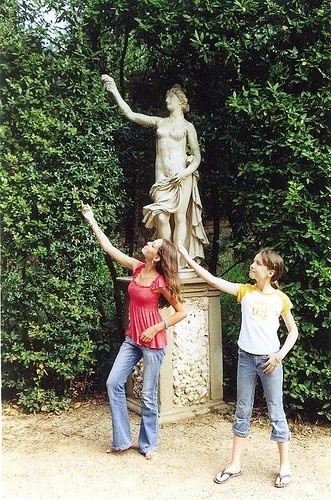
[175,242,299,487]
[100,73,210,269]
[81,203,190,461]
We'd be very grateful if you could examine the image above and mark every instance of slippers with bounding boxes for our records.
[274,472,291,488]
[215,469,242,484]
